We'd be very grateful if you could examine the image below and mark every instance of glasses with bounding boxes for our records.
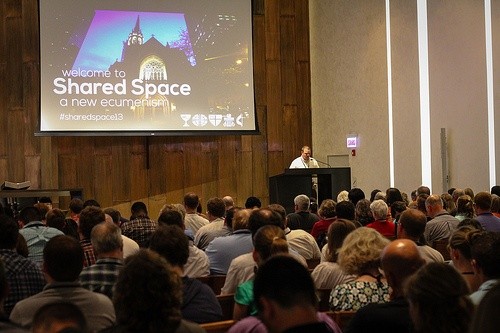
[182,201,185,205]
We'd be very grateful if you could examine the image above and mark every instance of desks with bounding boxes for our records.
[0,189,83,210]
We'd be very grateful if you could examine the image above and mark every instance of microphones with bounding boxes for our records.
[310,159,331,169]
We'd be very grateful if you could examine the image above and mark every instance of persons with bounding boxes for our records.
[289,145,320,169]
[0,186,500,333]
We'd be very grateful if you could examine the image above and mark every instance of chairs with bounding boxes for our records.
[200,274,357,333]
